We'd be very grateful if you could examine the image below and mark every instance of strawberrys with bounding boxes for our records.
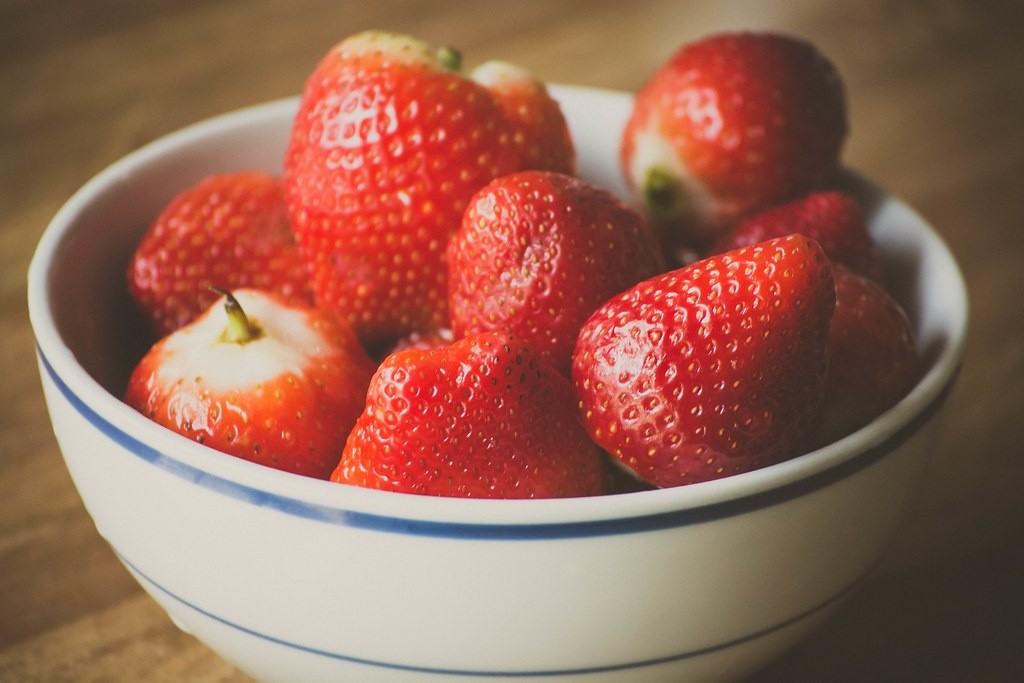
[123,30,917,501]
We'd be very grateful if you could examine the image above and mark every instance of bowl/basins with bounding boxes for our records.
[28,81,969,682]
